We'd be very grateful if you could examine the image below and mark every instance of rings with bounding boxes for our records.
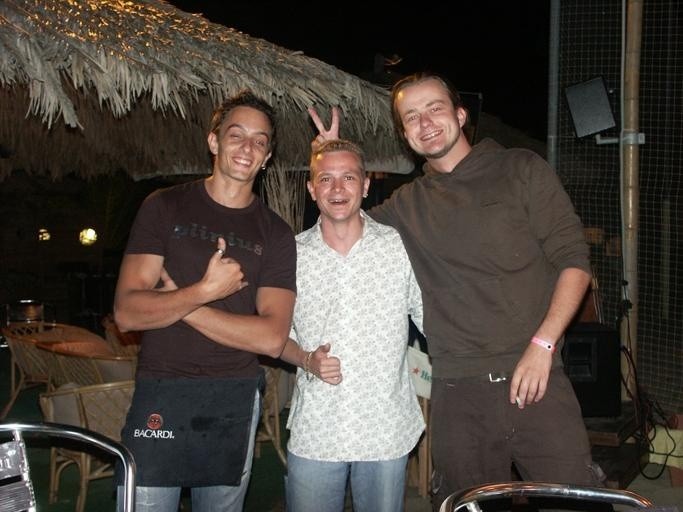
[215,249,225,256]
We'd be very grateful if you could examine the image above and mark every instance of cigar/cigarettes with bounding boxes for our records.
[515,394,522,406]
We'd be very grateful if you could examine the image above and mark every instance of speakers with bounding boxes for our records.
[562,320,620,418]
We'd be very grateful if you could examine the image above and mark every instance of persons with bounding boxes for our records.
[278,138,427,512]
[307,74,615,512]
[111,88,298,512]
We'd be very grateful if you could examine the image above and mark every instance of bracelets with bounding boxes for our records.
[305,351,314,382]
[530,335,556,353]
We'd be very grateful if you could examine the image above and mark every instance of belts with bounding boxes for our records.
[461,373,508,384]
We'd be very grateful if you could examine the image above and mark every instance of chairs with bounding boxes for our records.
[439,481,656,512]
